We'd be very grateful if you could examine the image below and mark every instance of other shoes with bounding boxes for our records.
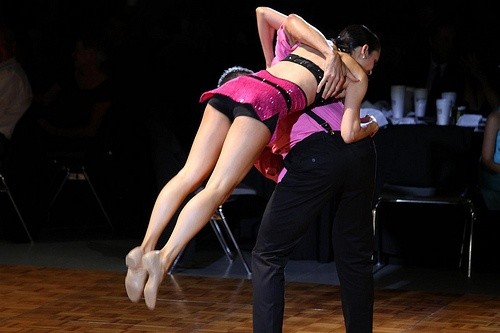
[143,250,165,311]
[125,246,149,303]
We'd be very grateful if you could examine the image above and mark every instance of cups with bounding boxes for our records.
[414,88,428,119]
[406,87,415,113]
[442,92,457,118]
[390,85,406,120]
[436,99,451,126]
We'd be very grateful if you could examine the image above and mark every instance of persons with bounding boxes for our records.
[124,7,383,308]
[49,38,112,138]
[478,109,500,210]
[217,12,381,333]
[0,31,33,140]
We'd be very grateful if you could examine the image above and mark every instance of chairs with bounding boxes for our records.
[163,149,264,277]
[370,123,475,281]
[0,137,118,247]
